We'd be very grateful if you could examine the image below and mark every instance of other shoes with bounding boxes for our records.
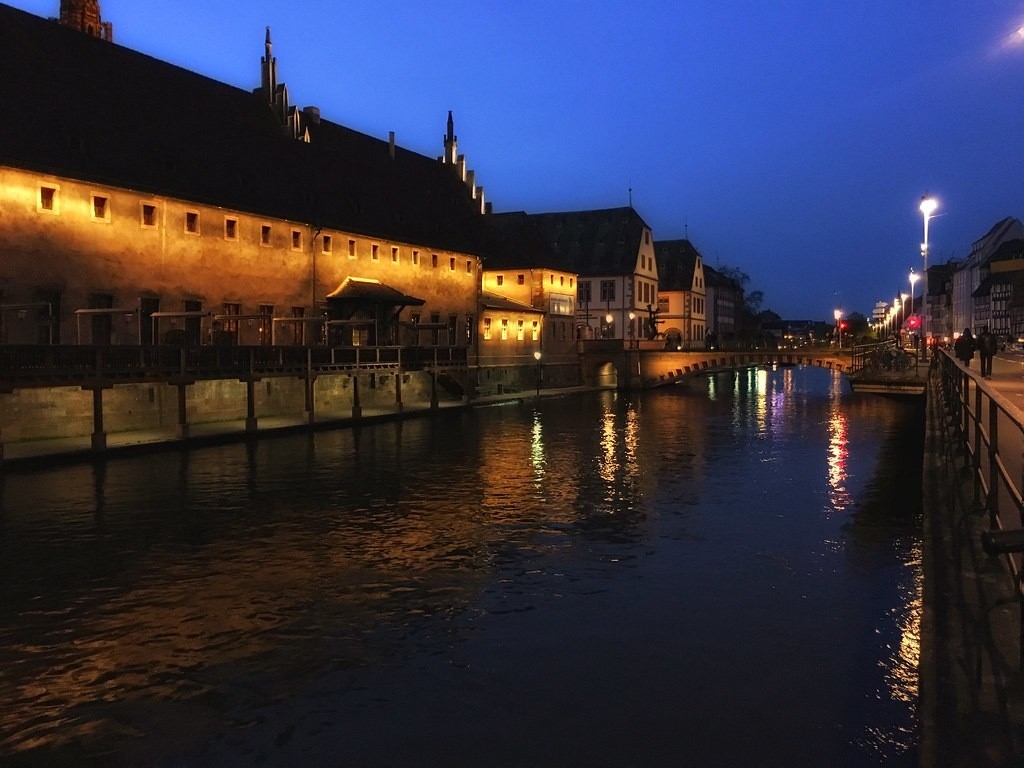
[988,374,992,380]
[983,377,985,381]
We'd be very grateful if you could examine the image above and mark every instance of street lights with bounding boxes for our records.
[884,306,898,336]
[909,274,916,314]
[533,352,541,396]
[834,310,841,348]
[607,314,611,338]
[902,295,905,324]
[921,196,932,362]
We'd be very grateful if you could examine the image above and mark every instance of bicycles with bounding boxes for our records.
[868,347,917,372]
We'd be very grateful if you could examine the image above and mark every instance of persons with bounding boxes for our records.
[207,320,222,345]
[975,325,997,380]
[954,327,977,369]
[705,327,713,352]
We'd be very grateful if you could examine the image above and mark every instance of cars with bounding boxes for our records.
[999,333,1013,338]
[996,339,1006,351]
[778,342,800,350]
